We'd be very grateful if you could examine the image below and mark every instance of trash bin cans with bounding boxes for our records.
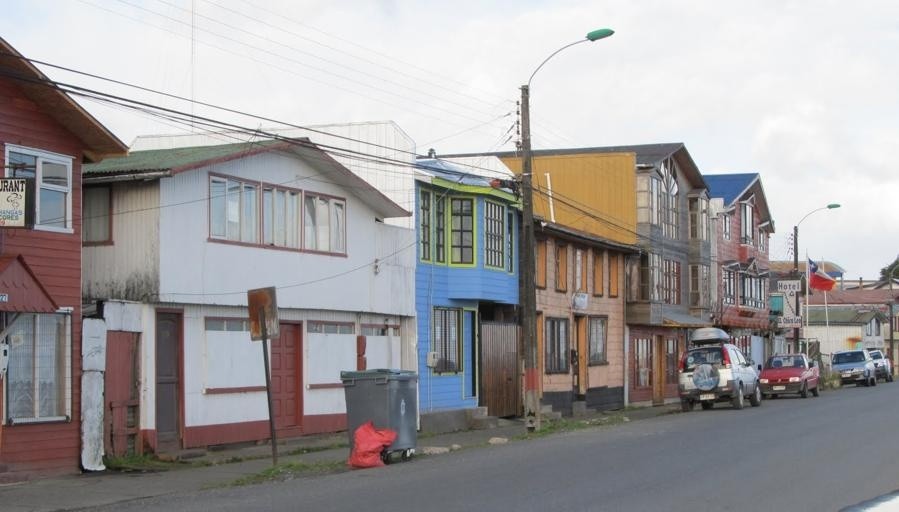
[339,369,420,465]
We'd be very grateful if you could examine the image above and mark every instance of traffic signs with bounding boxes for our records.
[515,28,617,436]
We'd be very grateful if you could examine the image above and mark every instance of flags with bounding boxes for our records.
[808,257,835,292]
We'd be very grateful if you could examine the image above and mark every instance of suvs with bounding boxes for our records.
[831,349,877,387]
[869,350,893,381]
[677,327,763,412]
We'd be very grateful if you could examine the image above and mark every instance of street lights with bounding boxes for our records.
[793,203,841,352]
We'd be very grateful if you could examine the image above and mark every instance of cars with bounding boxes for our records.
[757,352,821,397]
